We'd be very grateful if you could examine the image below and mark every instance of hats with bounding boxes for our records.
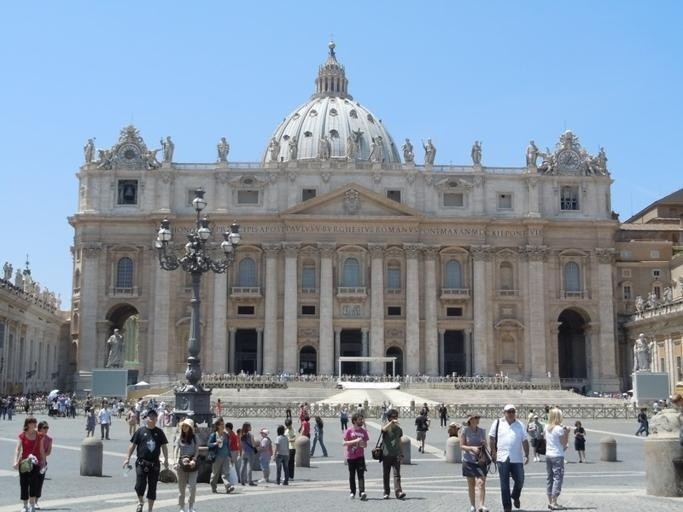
[465,413,481,423]
[142,408,157,418]
[504,404,516,410]
[178,418,194,432]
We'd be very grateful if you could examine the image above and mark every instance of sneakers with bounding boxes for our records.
[360,491,366,500]
[397,491,406,498]
[226,486,234,493]
[477,506,489,512]
[274,481,280,484]
[383,493,388,499]
[249,483,257,486]
[179,508,185,512]
[20,503,39,512]
[188,509,196,512]
[469,506,475,512]
[503,492,520,511]
[547,503,567,510]
[135,503,142,511]
[533,455,541,462]
[242,483,244,485]
[349,492,354,498]
[283,481,288,485]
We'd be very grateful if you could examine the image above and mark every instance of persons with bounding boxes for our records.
[381,409,406,499]
[472,140,481,166]
[488,404,529,512]
[269,135,436,166]
[160,136,174,161]
[173,419,199,512]
[343,414,369,501]
[636,395,683,436]
[543,407,568,510]
[217,137,230,161]
[122,409,168,512]
[340,402,461,453]
[598,145,608,171]
[526,404,588,465]
[581,154,604,177]
[459,413,489,512]
[104,329,124,368]
[539,148,558,175]
[13,417,53,512]
[83,138,96,164]
[526,140,539,170]
[634,333,652,370]
[206,417,295,493]
[1,392,171,440]
[216,398,222,416]
[286,402,327,457]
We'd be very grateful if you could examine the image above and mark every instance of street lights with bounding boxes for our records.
[152,188,242,423]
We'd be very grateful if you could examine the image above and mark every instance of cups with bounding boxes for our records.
[217,441,222,448]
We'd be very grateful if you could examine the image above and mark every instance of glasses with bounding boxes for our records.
[504,409,515,413]
[42,426,48,429]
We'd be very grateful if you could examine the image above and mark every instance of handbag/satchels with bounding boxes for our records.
[19,458,32,472]
[253,446,257,454]
[535,438,546,455]
[204,452,215,464]
[371,430,385,460]
[475,445,491,466]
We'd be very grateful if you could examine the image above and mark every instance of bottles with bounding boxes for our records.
[124,462,134,475]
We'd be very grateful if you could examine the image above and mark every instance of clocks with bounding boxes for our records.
[117,143,141,165]
[557,150,580,170]
